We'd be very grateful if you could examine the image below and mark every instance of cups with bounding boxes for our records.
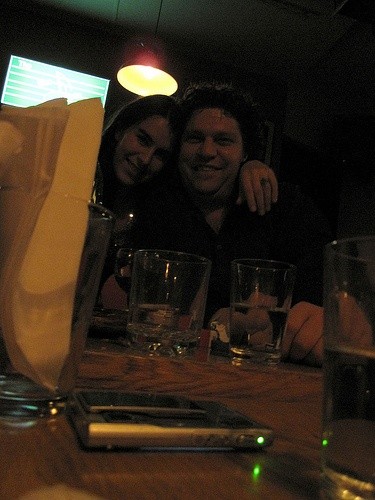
[315,234,375,499]
[112,247,138,294]
[125,247,213,361]
[230,258,298,371]
[0,201,115,424]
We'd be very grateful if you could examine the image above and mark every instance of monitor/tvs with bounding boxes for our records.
[0,53,112,115]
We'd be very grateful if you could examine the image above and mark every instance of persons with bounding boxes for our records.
[111,82,323,368]
[72,95,279,323]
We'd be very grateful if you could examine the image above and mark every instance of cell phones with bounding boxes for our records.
[76,390,208,418]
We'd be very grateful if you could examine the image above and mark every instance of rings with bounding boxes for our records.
[260,177,269,186]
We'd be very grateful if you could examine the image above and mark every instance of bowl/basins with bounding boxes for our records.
[87,307,130,339]
